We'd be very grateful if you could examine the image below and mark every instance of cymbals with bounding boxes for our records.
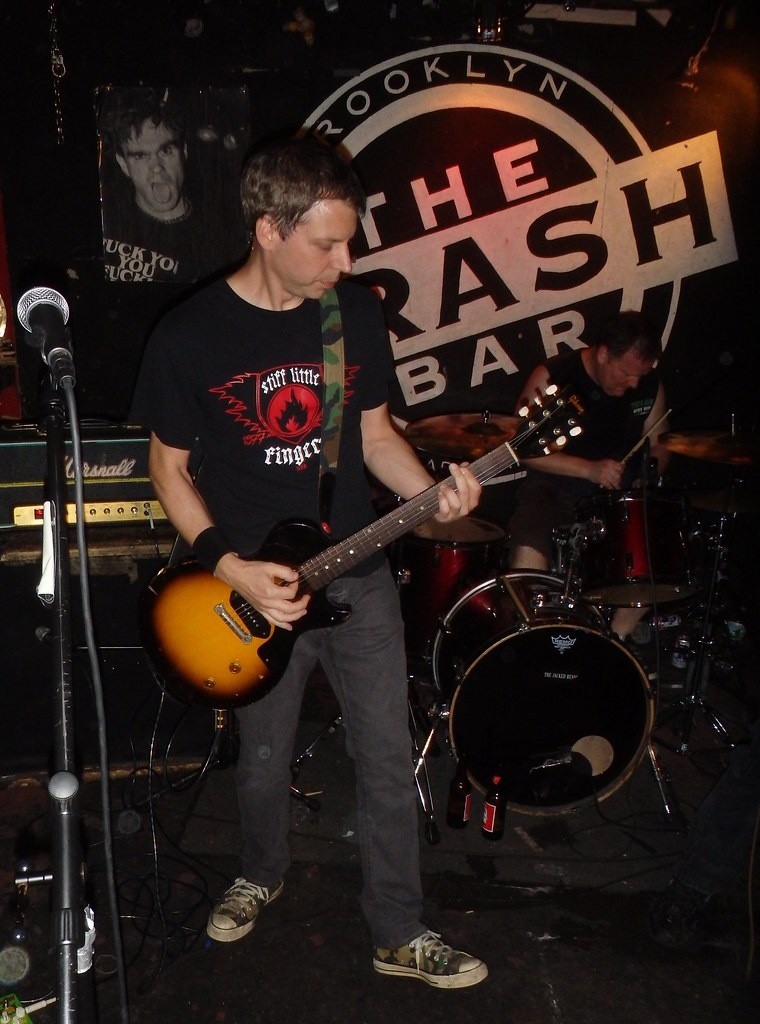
[403,413,571,462]
[656,429,760,468]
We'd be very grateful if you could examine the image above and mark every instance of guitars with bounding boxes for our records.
[132,384,587,711]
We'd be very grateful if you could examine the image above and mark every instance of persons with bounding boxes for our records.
[91,106,225,420]
[149,126,489,989]
[508,310,669,643]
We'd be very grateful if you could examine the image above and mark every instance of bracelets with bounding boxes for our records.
[193,526,239,576]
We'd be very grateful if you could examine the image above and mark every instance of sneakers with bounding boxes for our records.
[372,930,489,990]
[611,632,658,681]
[206,876,283,942]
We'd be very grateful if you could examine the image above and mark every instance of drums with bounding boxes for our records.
[381,514,513,692]
[426,565,656,818]
[576,577,704,716]
[587,480,698,586]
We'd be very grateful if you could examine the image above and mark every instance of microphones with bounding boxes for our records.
[34,626,99,663]
[17,287,76,388]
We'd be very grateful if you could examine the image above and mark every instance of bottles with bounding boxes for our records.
[481,775,507,840]
[672,606,692,670]
[635,613,683,630]
[446,752,472,829]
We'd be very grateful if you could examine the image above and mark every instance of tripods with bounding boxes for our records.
[653,475,736,756]
